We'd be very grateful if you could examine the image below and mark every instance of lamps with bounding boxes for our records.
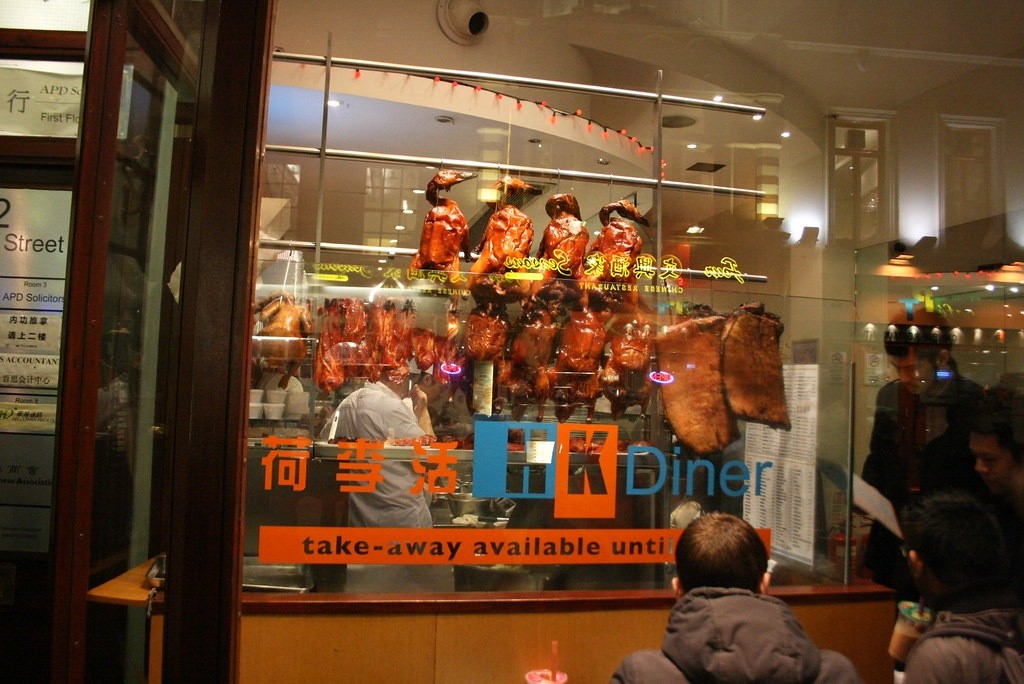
[683,221,705,235]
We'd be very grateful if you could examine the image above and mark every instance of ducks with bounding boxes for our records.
[409,168,478,311]
[448,177,653,424]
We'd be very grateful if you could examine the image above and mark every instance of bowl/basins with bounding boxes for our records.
[249,388,310,420]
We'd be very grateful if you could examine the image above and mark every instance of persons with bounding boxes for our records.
[255,360,303,393]
[852,304,1024,684]
[606,512,864,684]
[318,353,456,594]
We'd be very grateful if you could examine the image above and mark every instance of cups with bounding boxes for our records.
[888,600,935,661]
[525,669,568,684]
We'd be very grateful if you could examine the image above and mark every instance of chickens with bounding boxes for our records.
[251,290,316,390]
